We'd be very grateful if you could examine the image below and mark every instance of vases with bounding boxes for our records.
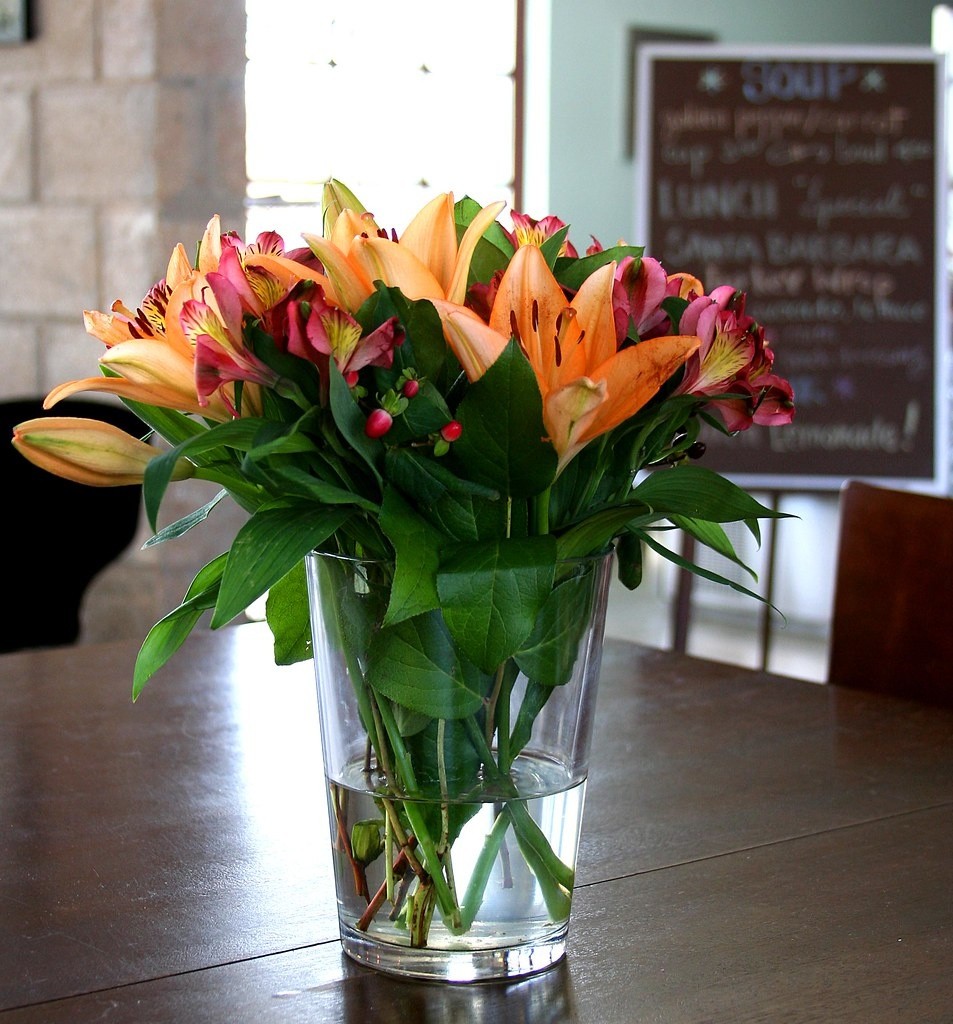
[302,547,616,982]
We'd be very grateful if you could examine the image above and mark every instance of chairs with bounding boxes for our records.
[0,395,151,651]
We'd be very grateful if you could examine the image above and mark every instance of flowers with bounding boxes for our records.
[8,177,800,941]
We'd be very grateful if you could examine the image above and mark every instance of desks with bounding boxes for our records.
[0,620,953,1024]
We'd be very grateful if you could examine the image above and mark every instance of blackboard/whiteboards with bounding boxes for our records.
[634,42,948,495]
